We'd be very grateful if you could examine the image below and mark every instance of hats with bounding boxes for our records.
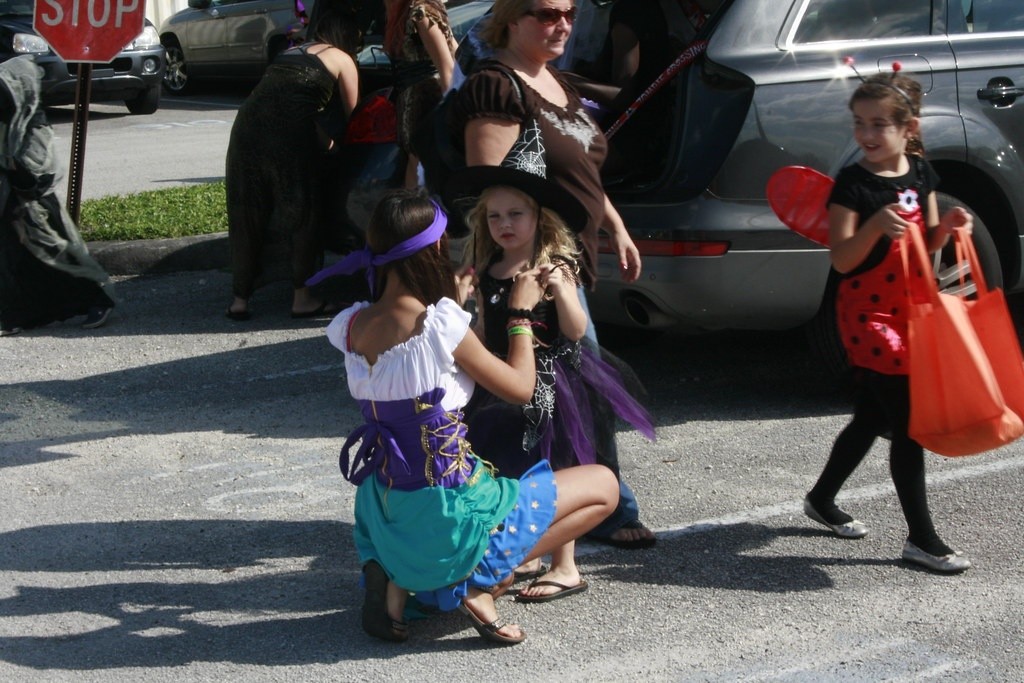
[446,118,587,233]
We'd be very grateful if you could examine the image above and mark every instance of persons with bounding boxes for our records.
[225,7,359,320]
[461,0,657,550]
[324,188,620,643]
[382,0,467,189]
[448,116,588,603]
[803,72,973,572]
[0,54,117,336]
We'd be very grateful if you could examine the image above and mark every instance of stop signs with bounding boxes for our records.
[32,0,145,63]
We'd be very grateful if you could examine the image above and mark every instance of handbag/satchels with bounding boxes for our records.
[410,64,525,237]
[898,223,1024,457]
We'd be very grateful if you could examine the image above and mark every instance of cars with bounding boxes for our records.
[0,0,165,115]
[158,1,316,97]
[338,0,493,235]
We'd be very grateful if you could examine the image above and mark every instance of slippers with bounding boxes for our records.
[292,300,340,318]
[508,565,546,579]
[516,577,589,603]
[227,305,251,320]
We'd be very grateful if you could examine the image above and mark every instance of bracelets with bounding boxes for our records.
[506,307,535,336]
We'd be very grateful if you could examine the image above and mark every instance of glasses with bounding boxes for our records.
[524,6,578,25]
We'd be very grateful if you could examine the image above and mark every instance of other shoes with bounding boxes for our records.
[901,536,972,572]
[0,321,19,336]
[804,492,869,538]
[82,305,111,327]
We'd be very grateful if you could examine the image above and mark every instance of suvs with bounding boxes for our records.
[431,0,1024,389]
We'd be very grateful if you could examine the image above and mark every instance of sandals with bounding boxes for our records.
[457,583,526,642]
[362,559,409,641]
[586,519,656,548]
